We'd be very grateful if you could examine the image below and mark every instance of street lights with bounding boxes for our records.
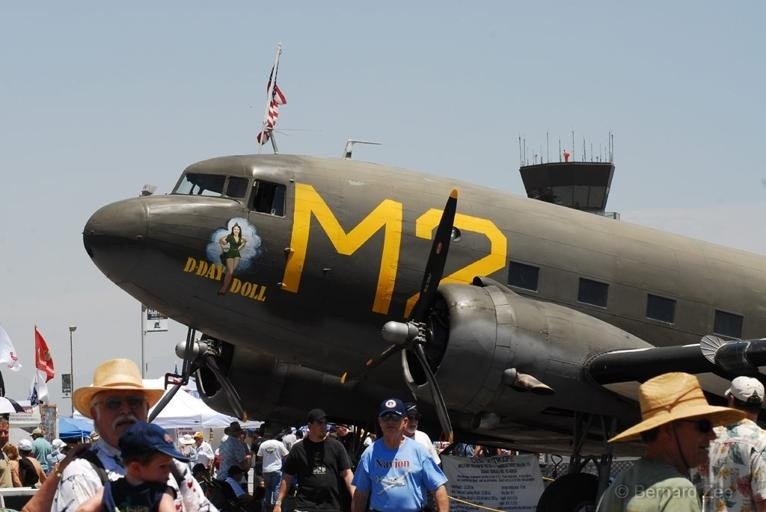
[68,325,76,414]
[139,300,168,379]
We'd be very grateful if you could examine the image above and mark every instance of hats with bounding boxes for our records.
[378,397,406,419]
[608,372,747,445]
[70,358,165,421]
[224,421,243,437]
[228,465,247,474]
[192,463,209,476]
[117,422,191,463]
[402,400,422,417]
[306,408,327,424]
[17,427,68,451]
[721,374,766,405]
[178,431,204,445]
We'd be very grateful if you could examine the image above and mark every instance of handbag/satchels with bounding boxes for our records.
[20,442,92,512]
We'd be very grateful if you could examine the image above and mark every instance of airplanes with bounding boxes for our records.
[78,43,766,459]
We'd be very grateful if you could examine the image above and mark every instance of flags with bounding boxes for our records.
[257,61,287,145]
[0,326,23,372]
[34,326,55,383]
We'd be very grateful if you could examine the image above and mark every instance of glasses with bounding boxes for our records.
[381,414,401,423]
[92,394,147,411]
[681,415,713,435]
[407,415,420,422]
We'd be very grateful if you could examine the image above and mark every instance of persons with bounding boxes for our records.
[452,442,520,457]
[595,372,748,512]
[1,359,218,510]
[687,375,766,512]
[217,222,247,296]
[213,397,449,512]
